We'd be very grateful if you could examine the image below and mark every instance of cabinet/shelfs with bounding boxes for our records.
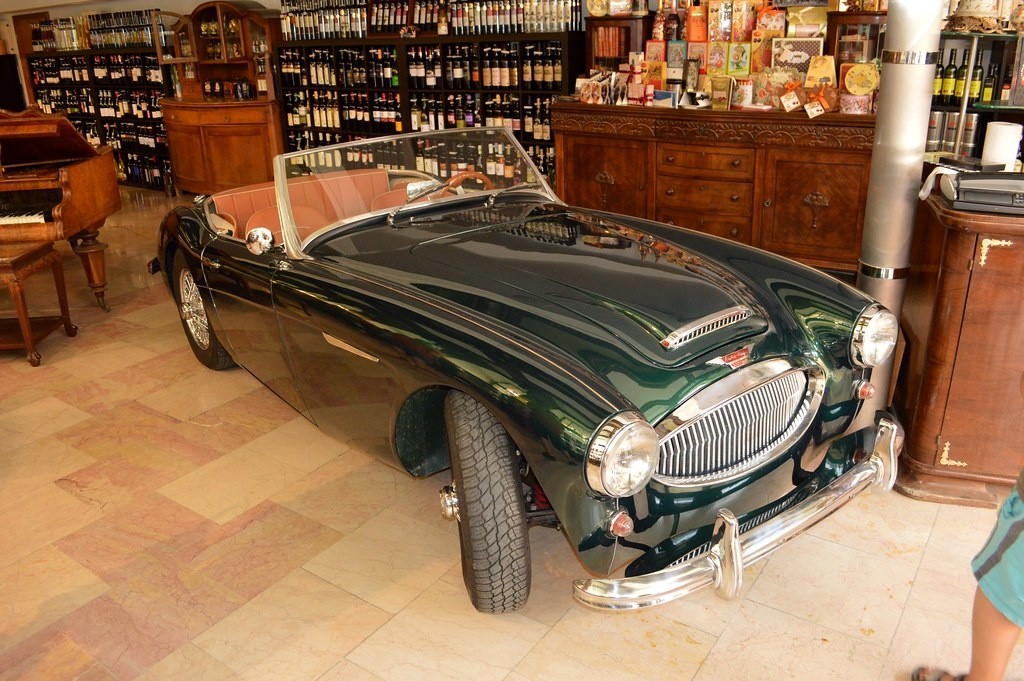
[25,46,176,191]
[159,0,282,196]
[273,31,585,190]
[550,103,876,273]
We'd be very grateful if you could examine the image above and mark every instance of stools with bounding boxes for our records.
[0,241,78,367]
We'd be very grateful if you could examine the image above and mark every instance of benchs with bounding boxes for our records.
[204,169,446,239]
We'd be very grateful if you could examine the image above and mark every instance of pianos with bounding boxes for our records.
[0,106,123,313]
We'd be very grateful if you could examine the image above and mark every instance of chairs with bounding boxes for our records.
[371,189,455,212]
[245,204,329,245]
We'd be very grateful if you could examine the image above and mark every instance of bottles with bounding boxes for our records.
[632,0,648,16]
[652,0,707,43]
[982,61,1015,102]
[953,48,968,106]
[942,48,958,106]
[274,0,583,192]
[932,48,944,105]
[204,38,268,101]
[967,48,984,108]
[22,9,175,189]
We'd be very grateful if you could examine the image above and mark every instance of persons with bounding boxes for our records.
[911,466,1024,681]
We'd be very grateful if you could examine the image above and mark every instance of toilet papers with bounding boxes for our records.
[981,121,1023,172]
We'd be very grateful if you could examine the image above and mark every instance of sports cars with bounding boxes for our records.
[145,120,911,616]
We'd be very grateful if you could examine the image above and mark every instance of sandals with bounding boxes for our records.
[910,665,968,681]
